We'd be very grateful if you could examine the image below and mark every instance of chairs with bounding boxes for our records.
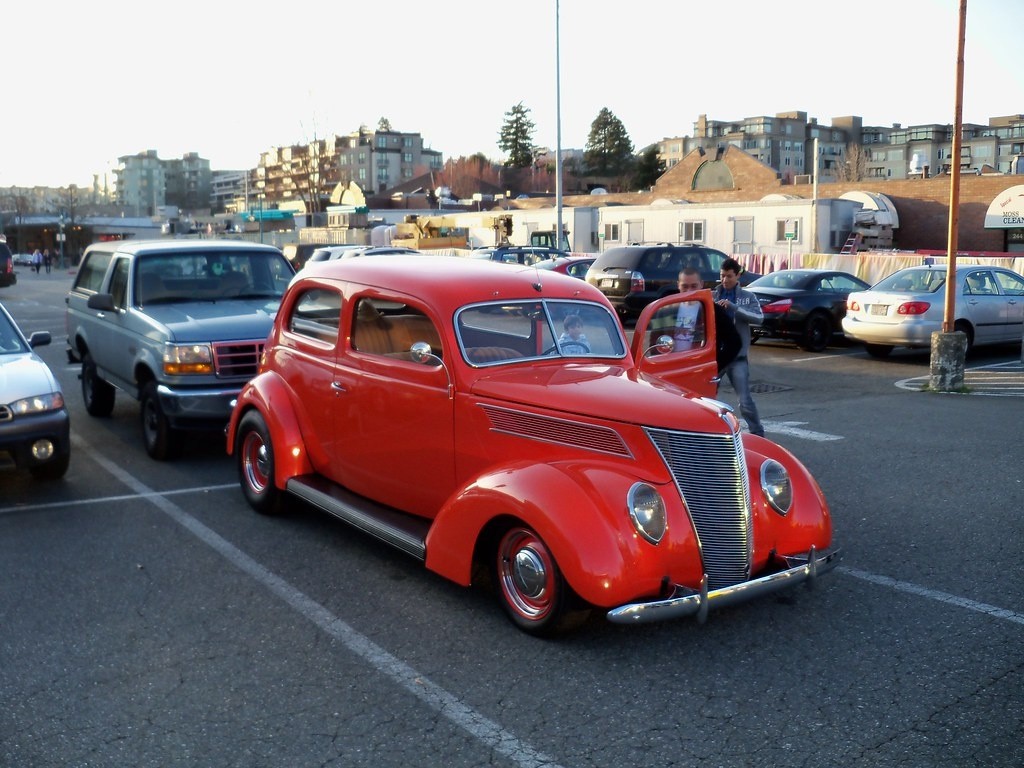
[216,271,251,296]
[894,278,912,290]
[776,277,791,287]
[465,347,525,363]
[136,273,168,303]
[966,276,986,294]
[382,353,439,367]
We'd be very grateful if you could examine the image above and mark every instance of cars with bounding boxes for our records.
[228,254,840,639]
[525,257,596,281]
[0,242,17,288]
[14,254,33,265]
[0,303,71,491]
[64,238,297,464]
[586,242,765,329]
[476,245,569,268]
[841,258,1024,359]
[743,269,871,352]
[280,244,422,274]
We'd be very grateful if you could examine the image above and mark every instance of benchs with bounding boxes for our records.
[312,315,443,353]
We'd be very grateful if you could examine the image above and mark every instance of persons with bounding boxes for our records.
[648,257,766,437]
[549,314,592,356]
[32,248,52,274]
